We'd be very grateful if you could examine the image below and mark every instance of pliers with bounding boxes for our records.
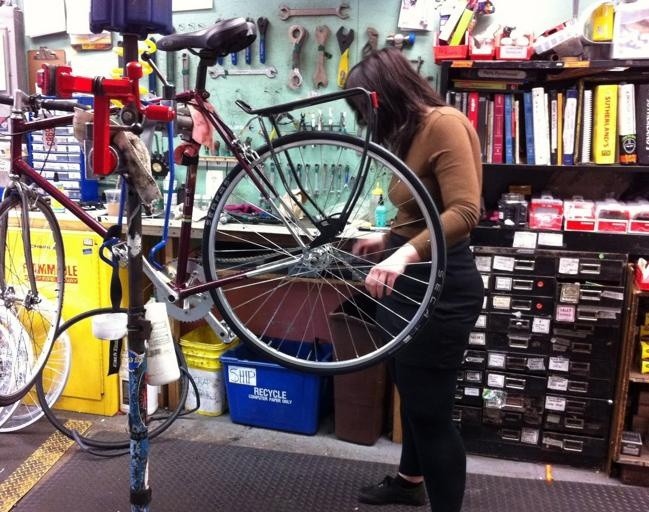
[298,112,308,149]
[337,113,348,151]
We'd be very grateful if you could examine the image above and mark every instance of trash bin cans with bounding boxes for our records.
[329,290,388,446]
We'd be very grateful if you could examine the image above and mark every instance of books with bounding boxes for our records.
[440,68,649,168]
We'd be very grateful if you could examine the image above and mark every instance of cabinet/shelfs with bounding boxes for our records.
[440,59,649,256]
[604,256,649,488]
[452,244,628,469]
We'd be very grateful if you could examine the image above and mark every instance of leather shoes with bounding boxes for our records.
[356,475,425,506]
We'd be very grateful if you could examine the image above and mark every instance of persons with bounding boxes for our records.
[342,47,484,512]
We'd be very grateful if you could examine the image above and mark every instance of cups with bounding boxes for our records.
[103,188,124,215]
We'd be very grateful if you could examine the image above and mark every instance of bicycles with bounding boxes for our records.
[0,16,449,404]
[0,282,73,434]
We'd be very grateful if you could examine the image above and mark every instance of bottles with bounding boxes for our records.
[370,182,387,226]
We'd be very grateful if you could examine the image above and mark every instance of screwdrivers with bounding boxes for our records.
[205,137,252,178]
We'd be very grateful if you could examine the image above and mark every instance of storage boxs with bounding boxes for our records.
[620,390,649,458]
[177,324,335,434]
